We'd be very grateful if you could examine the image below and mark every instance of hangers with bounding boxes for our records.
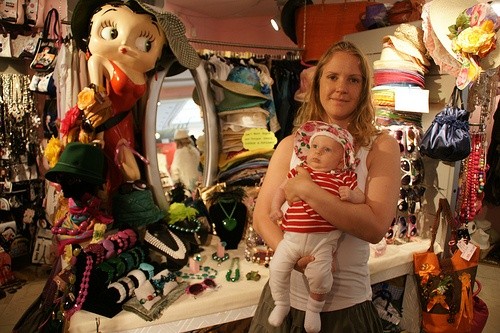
[190,38,300,60]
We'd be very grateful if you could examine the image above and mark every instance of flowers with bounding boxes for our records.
[448,13,497,58]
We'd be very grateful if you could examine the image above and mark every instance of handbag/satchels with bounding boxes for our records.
[421,85,471,163]
[372,282,404,300]
[0,210,30,258]
[27,8,64,95]
[27,187,74,264]
[413,197,488,333]
[371,293,401,330]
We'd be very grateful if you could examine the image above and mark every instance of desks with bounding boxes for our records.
[67,227,443,333]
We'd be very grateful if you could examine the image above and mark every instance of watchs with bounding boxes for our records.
[84,229,155,303]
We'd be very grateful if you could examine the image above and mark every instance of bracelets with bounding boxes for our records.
[212,252,229,262]
[139,290,162,304]
[155,274,175,288]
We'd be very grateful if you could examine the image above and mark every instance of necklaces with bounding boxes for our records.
[64,255,93,318]
[218,201,237,232]
[226,257,240,281]
[454,134,487,230]
[169,217,202,233]
[143,230,187,260]
[245,244,273,264]
[64,255,90,315]
[0,73,41,156]
[174,265,218,280]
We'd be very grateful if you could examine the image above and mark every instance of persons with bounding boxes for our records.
[244,42,401,333]
[169,129,200,192]
[60,0,201,181]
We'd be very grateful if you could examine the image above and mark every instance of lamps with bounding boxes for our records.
[270,2,284,32]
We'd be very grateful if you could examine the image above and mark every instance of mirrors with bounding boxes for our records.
[143,58,219,214]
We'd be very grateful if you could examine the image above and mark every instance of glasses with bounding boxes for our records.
[395,130,404,154]
[385,217,395,239]
[185,278,216,299]
[409,215,417,238]
[400,158,412,184]
[399,216,408,239]
[398,186,426,213]
[412,158,425,185]
[406,128,414,153]
[76,110,97,134]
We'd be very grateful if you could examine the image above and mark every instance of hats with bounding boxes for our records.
[293,121,355,171]
[43,142,109,183]
[293,66,317,102]
[196,136,208,151]
[125,0,201,76]
[469,228,491,250]
[171,130,189,140]
[210,66,276,185]
[369,22,430,122]
[111,180,171,232]
